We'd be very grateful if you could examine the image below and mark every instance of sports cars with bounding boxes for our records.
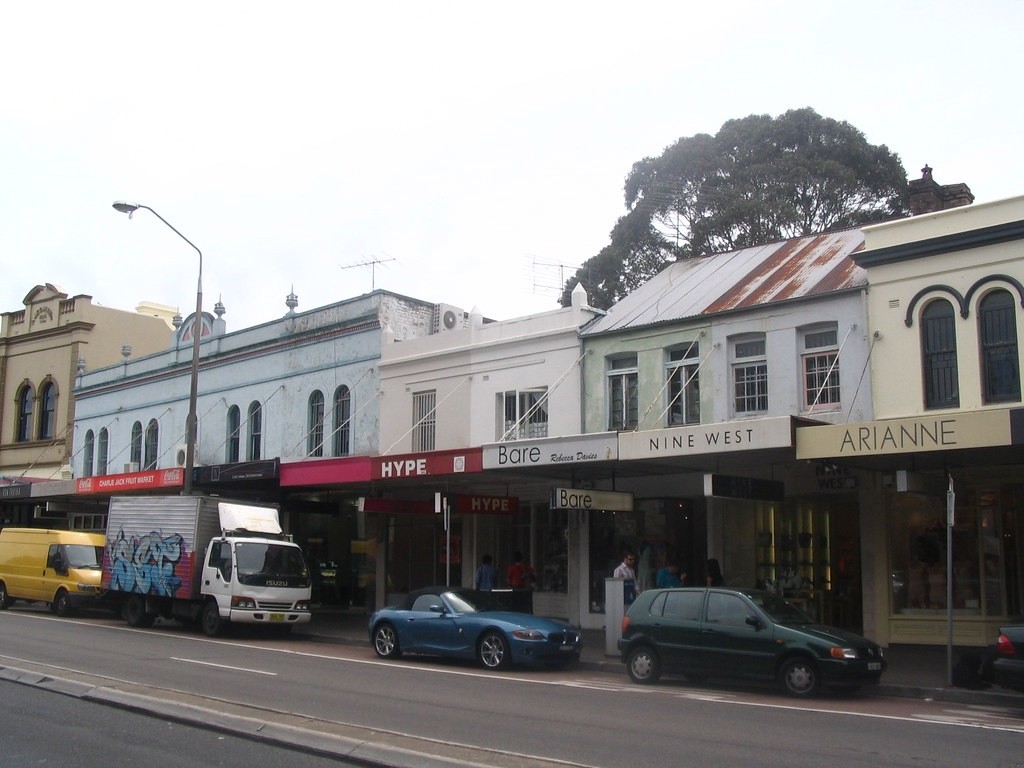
[368,585,584,671]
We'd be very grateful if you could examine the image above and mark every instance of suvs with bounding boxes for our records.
[617,585,888,699]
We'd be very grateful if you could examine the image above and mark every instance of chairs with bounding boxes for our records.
[261,558,284,574]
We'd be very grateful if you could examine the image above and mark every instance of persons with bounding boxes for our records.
[475,555,496,591]
[706,558,725,586]
[657,560,692,589]
[614,551,641,614]
[507,552,527,588]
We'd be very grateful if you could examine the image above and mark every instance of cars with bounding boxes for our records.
[993,625,1024,694]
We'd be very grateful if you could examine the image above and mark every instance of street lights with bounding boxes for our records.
[111,200,204,496]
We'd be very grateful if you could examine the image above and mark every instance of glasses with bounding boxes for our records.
[626,556,633,561]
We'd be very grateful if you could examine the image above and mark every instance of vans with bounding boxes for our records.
[0,527,106,618]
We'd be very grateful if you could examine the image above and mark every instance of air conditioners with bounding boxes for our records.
[433,303,464,332]
[123,462,139,473]
[176,444,187,468]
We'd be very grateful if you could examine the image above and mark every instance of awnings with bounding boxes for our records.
[0,406,1024,499]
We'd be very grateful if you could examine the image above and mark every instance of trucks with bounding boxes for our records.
[100,495,313,639]
